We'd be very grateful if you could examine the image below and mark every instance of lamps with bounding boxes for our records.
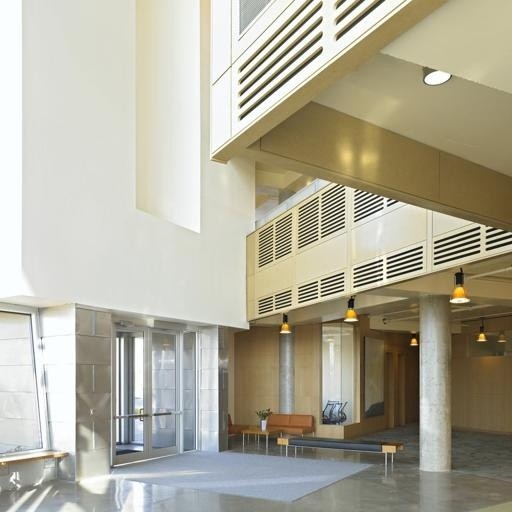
[449,268,471,304]
[280,313,292,334]
[344,295,359,322]
[409,334,418,346]
[476,318,489,341]
[423,66,452,85]
[496,330,507,342]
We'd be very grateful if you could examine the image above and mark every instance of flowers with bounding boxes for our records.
[256,409,272,420]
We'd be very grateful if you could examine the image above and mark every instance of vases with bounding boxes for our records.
[260,419,266,432]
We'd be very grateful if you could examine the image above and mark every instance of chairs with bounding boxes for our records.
[228,416,247,439]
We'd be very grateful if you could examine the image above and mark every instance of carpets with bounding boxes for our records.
[110,450,373,503]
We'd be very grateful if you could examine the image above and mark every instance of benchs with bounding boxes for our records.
[277,437,404,463]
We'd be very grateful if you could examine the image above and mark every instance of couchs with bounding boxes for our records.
[249,414,315,437]
